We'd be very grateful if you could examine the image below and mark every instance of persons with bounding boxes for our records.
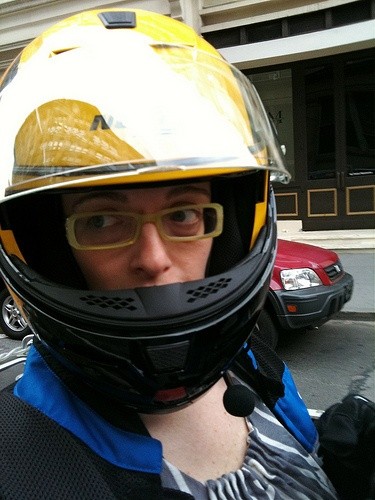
[0,7,344,500]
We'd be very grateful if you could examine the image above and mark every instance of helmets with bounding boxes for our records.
[2,8,277,417]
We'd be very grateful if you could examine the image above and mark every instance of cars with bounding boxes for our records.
[0,237,355,352]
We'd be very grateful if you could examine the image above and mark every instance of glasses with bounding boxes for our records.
[53,201,224,250]
